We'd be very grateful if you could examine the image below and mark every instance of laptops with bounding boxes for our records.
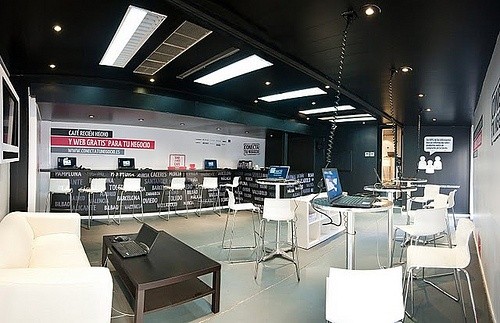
[374,167,400,188]
[257,165,290,182]
[322,168,375,208]
[118,158,138,170]
[111,222,160,258]
[205,160,222,170]
[57,157,78,170]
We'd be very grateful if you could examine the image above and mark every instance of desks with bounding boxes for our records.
[311,176,460,269]
[256,177,298,262]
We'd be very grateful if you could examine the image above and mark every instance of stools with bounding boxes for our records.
[158,177,189,222]
[323,185,479,323]
[45,177,73,213]
[214,176,240,215]
[75,178,112,231]
[221,188,262,263]
[112,177,144,225]
[254,198,300,283]
[195,177,222,217]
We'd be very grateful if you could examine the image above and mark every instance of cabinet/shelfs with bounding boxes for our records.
[288,192,348,250]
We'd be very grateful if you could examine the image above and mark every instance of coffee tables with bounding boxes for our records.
[102,231,223,322]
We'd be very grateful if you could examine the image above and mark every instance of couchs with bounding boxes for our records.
[1,211,114,322]
